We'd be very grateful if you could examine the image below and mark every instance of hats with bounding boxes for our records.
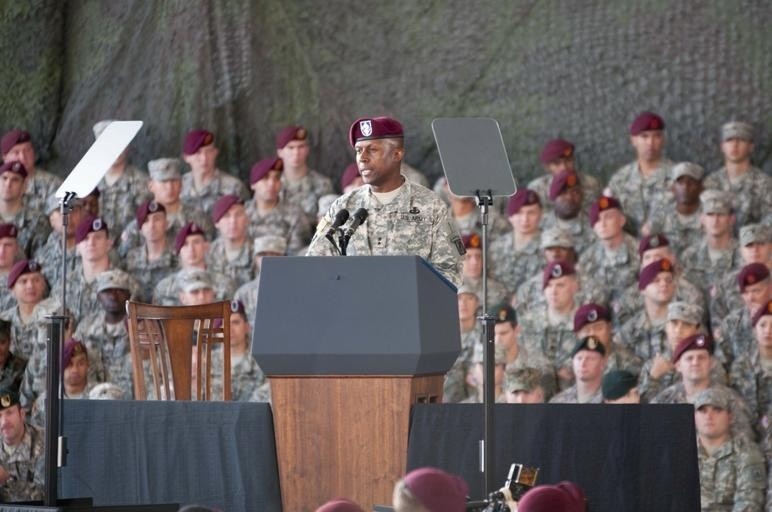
[549,169,580,200]
[489,301,772,409]
[507,188,539,215]
[349,117,404,148]
[543,260,576,288]
[740,223,770,246]
[458,282,478,297]
[540,140,574,165]
[460,232,482,249]
[703,198,732,214]
[722,121,755,140]
[541,230,574,248]
[672,162,705,181]
[136,126,307,332]
[590,196,621,227]
[639,234,669,262]
[315,498,363,512]
[630,112,664,135]
[341,164,359,189]
[738,263,770,286]
[519,482,583,511]
[639,258,674,289]
[0,119,129,411]
[400,469,468,512]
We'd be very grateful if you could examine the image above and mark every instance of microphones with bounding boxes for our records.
[345,207,370,244]
[327,208,349,239]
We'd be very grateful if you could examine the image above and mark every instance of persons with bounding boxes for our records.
[0,116,771,511]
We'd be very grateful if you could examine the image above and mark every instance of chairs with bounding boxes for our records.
[125,300,231,400]
